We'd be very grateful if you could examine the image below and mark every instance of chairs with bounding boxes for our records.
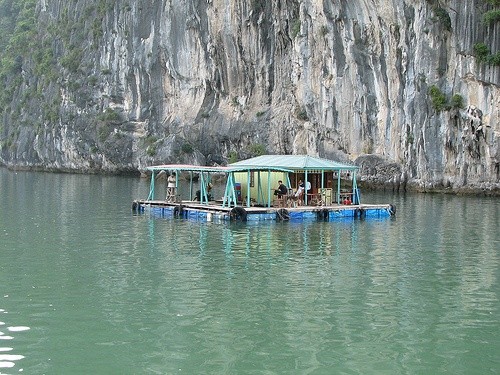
[272,188,356,209]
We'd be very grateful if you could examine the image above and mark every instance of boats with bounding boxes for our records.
[131,153,397,224]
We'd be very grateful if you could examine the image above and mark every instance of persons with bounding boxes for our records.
[306,179,311,204]
[166,171,176,203]
[295,179,304,205]
[270,180,288,200]
